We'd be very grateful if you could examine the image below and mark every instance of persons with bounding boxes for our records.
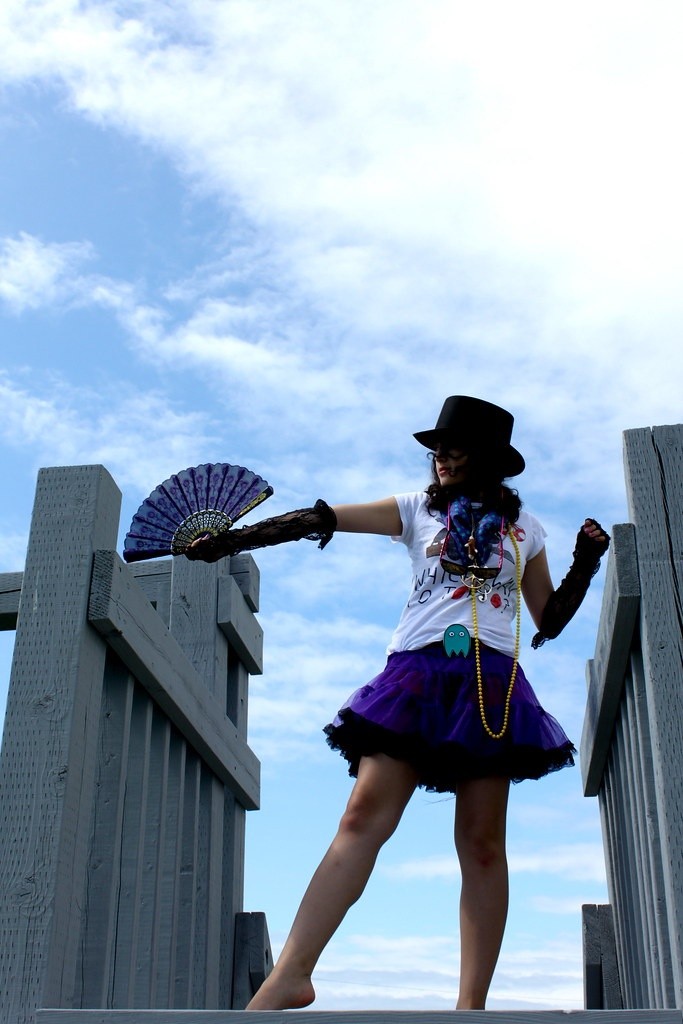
[184,393,611,1013]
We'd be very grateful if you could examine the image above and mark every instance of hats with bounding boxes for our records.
[413,396,525,478]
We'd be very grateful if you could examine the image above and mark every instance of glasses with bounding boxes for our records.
[440,529,504,579]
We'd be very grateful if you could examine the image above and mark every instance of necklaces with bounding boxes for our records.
[458,481,522,740]
